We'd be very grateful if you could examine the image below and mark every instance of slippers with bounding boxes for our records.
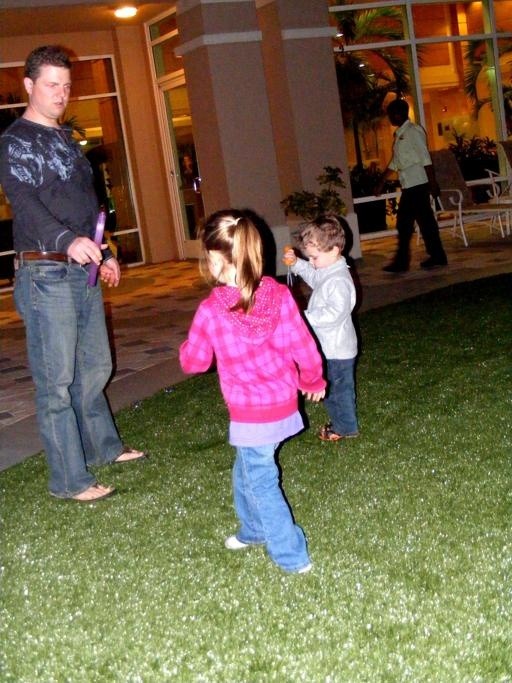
[71,483,117,503]
[114,448,146,464]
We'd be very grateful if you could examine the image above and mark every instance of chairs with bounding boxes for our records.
[426,147,512,247]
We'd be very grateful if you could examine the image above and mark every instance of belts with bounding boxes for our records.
[16,251,68,263]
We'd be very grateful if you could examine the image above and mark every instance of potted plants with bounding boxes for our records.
[328,3,415,234]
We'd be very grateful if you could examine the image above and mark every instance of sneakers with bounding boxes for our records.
[225,536,247,550]
[318,424,359,441]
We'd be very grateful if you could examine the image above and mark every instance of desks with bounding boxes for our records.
[462,173,512,190]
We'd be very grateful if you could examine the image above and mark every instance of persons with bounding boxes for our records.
[283,215,358,441]
[0,45,144,501]
[179,210,328,574]
[374,99,448,272]
